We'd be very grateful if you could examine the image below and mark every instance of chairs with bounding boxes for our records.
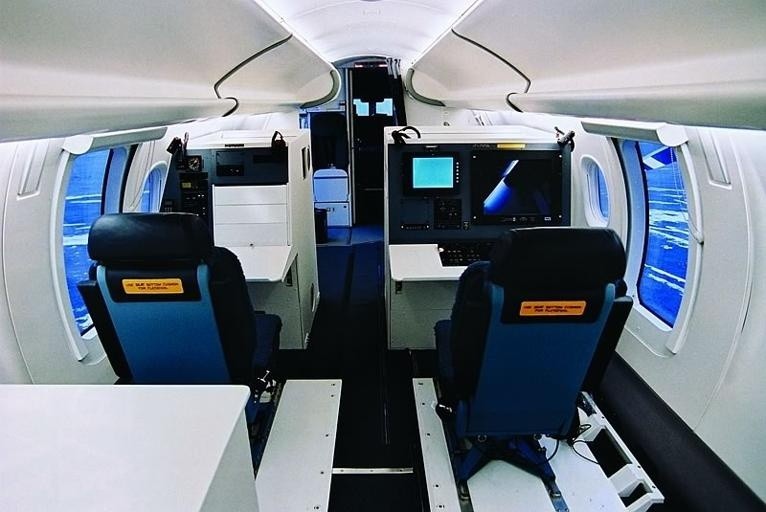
[430,227,634,499]
[77,212,282,432]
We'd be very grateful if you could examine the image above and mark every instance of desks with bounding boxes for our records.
[0,385,258,512]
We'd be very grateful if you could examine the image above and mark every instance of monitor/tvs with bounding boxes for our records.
[468,143,572,228]
[402,152,462,197]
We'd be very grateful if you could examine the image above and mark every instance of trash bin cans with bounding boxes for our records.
[314,208,329,243]
